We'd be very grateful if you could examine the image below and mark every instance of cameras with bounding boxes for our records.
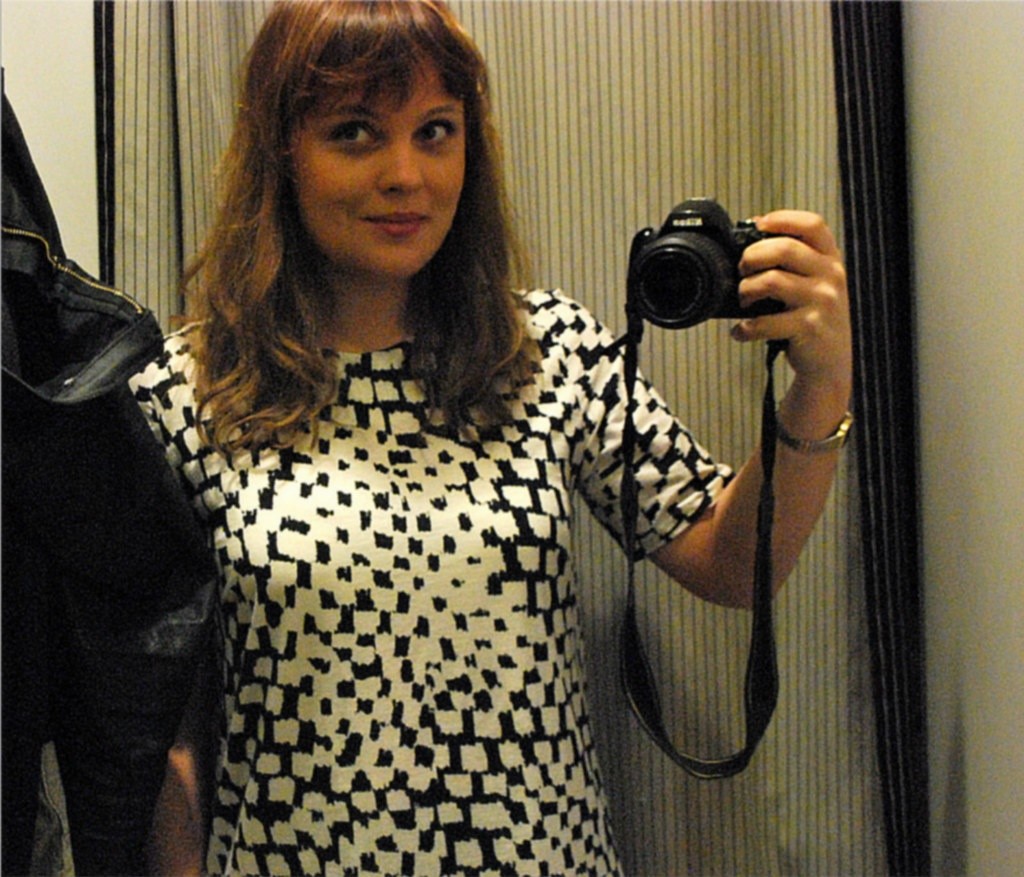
[627,197,787,330]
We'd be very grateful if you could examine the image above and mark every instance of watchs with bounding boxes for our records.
[776,405,853,452]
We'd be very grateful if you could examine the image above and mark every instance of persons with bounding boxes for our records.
[133,0,851,877]
